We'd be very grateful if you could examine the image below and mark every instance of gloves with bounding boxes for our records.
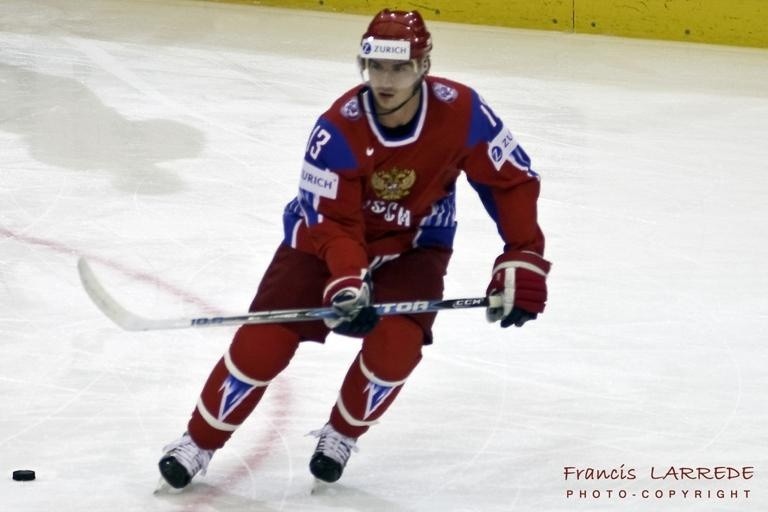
[321,267,379,337]
[486,251,551,328]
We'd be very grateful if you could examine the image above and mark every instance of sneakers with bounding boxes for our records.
[312,420,357,482]
[159,431,215,488]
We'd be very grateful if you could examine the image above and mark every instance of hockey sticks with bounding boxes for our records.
[77,259,504,331]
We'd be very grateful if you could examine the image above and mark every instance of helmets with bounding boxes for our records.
[357,9,432,90]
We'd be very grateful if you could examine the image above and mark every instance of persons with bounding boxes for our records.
[150,9,551,495]
